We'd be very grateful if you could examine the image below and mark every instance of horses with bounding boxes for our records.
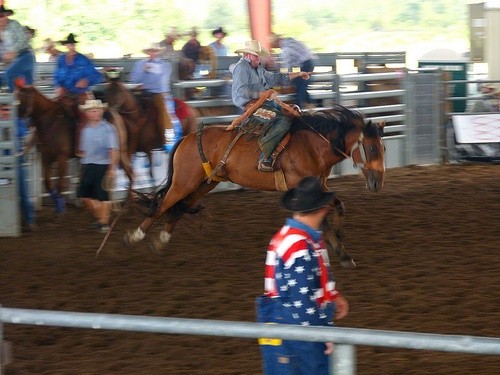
[175,64,240,119]
[119,100,389,269]
[96,71,204,203]
[11,82,139,224]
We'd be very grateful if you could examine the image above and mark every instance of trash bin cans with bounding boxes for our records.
[418,59,468,112]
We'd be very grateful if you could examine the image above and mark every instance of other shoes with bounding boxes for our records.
[258,158,275,173]
[23,223,40,233]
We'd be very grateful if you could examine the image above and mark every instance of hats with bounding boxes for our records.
[61,33,79,44]
[269,30,287,46]
[212,26,227,37]
[24,26,34,33]
[77,99,109,111]
[233,38,264,57]
[278,174,337,213]
[0,6,14,16]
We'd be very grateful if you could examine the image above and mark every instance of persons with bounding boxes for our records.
[0,5,103,98]
[0,102,39,232]
[231,39,315,171]
[128,25,227,169]
[479,83,500,112]
[266,32,318,110]
[255,177,348,375]
[76,101,121,232]
